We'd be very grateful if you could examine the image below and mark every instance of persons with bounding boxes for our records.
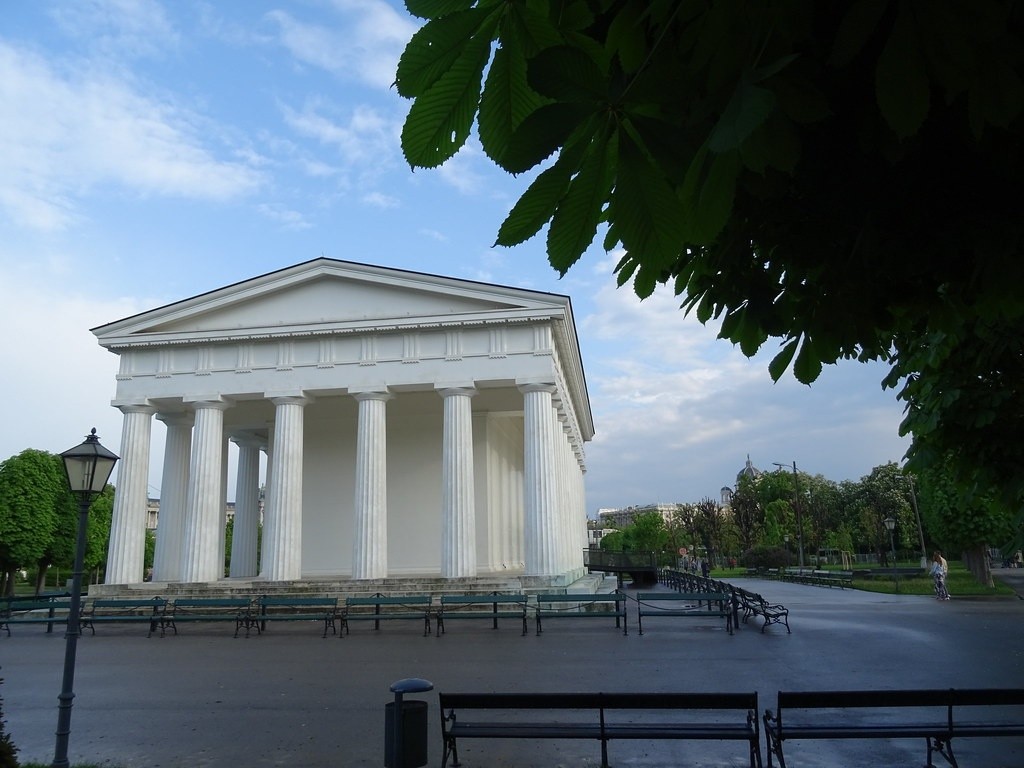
[1014,549,1023,568]
[684,556,710,577]
[928,551,951,601]
[730,558,737,570]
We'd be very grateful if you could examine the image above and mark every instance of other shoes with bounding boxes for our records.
[947,595,951,597]
[945,598,949,600]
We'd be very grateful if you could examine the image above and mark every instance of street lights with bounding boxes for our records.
[50,427,123,767]
[893,474,927,557]
[883,517,900,593]
[771,461,804,568]
[783,533,792,568]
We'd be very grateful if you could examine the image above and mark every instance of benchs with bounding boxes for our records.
[437,691,765,768]
[80,599,168,637]
[743,568,854,591]
[34,591,88,605]
[247,597,338,639]
[0,601,85,639]
[636,592,734,635]
[160,599,252,639]
[656,569,792,636]
[338,596,432,638]
[435,595,529,638]
[764,686,1024,768]
[536,594,629,636]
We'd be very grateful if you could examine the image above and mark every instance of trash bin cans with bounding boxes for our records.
[384,679,433,768]
